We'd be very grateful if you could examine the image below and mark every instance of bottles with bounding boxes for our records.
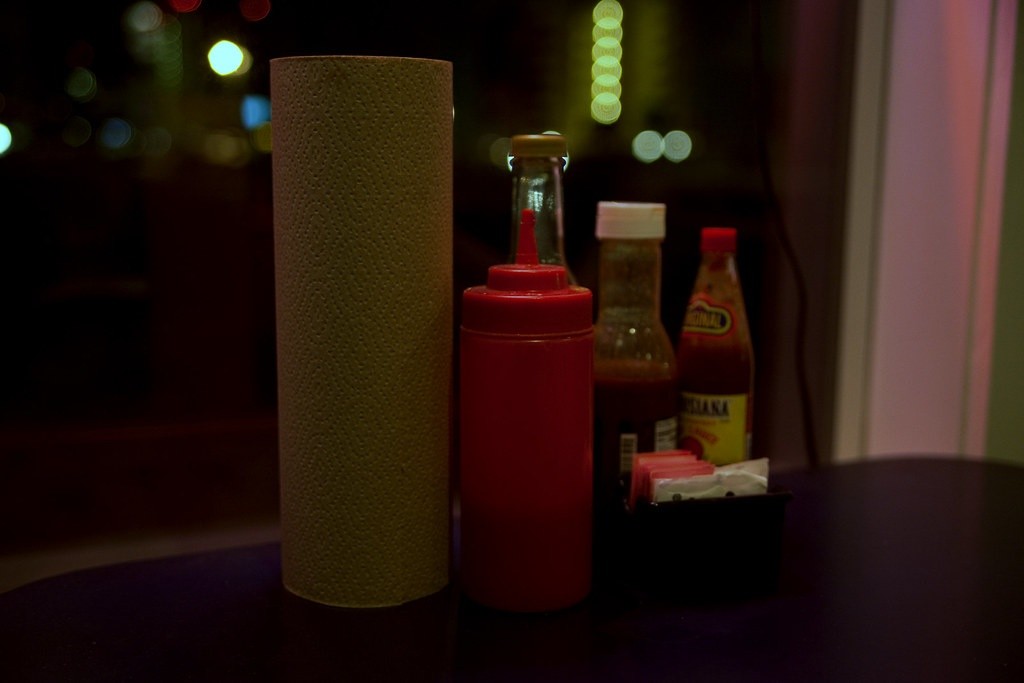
[592,202,682,560]
[504,136,577,287]
[461,211,593,613]
[680,227,754,464]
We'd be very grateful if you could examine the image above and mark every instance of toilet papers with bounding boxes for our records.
[269,58,454,610]
